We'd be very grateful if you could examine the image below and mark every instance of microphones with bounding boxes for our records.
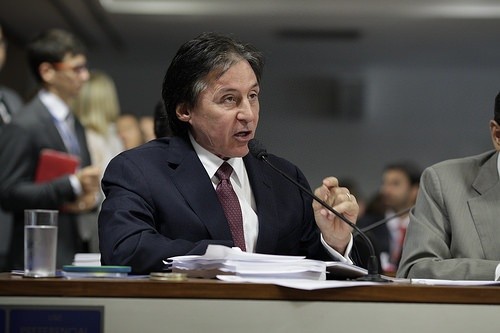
[248,137,392,283]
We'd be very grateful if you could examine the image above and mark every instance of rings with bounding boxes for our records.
[345,192,352,201]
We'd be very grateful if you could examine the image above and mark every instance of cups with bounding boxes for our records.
[23,209,58,278]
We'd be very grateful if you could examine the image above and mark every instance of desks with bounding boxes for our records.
[0,271,500,333]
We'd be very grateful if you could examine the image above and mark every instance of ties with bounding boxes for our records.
[214,161,246,252]
[388,226,407,275]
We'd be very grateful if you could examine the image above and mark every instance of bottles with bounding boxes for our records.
[71,252,101,267]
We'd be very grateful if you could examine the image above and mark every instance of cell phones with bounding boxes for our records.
[62,265,131,272]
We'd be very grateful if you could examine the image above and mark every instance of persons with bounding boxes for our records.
[353,159,426,271]
[99,32,374,272]
[396,93,500,282]
[0,26,154,265]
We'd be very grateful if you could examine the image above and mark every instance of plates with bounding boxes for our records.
[61,265,131,280]
